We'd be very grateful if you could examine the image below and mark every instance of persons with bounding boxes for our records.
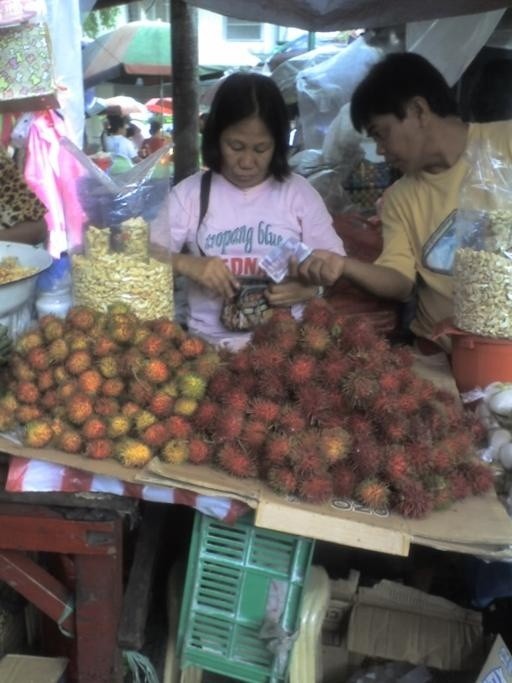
[286,48,511,365]
[146,69,346,355]
[101,109,211,166]
[1,141,51,246]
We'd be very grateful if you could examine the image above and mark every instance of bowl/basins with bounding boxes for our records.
[0,240,54,315]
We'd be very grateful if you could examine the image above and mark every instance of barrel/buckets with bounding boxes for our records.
[432,316,512,393]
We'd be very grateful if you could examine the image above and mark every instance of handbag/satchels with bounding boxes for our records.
[220,277,292,331]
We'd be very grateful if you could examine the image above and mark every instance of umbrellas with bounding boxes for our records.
[81,17,261,131]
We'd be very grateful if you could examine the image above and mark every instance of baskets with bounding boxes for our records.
[175,509,317,683]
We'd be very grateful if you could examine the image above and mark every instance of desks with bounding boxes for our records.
[0,500,143,683]
